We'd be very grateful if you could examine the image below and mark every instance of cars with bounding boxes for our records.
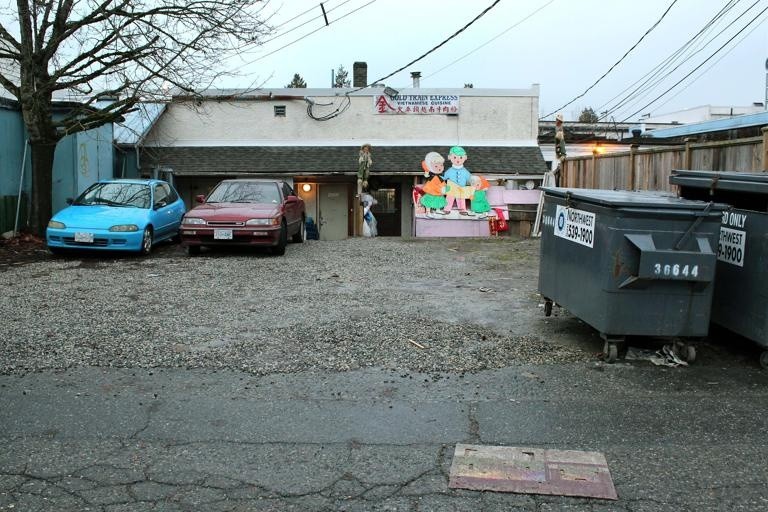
[178,177,309,258]
[43,177,186,255]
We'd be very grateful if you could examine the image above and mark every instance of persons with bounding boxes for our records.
[356,143,375,198]
[362,192,377,237]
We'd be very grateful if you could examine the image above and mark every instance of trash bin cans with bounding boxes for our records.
[668,170,767,369]
[538,185,730,368]
